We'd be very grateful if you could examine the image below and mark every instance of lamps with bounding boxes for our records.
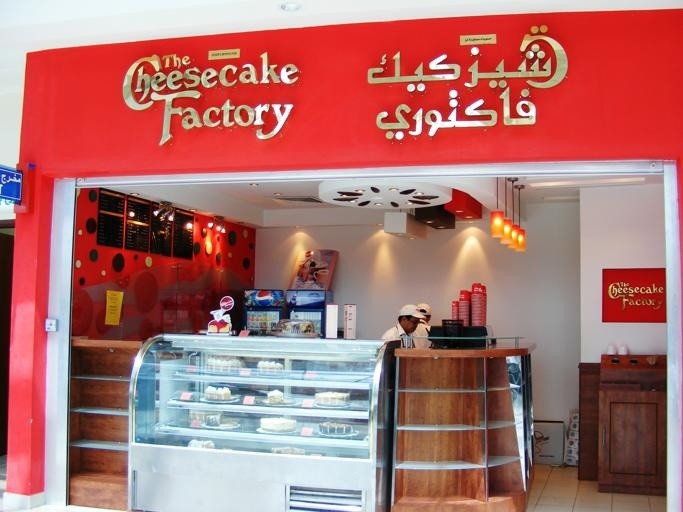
[490,177,526,252]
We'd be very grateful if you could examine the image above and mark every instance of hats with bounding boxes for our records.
[397,303,431,318]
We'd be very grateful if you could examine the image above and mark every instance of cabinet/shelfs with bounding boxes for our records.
[388,343,532,512]
[127,336,401,512]
[68,334,147,512]
[598,388,666,496]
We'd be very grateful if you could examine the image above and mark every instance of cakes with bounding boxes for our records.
[188,358,356,454]
[281,320,313,334]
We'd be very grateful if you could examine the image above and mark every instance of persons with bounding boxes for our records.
[381,303,433,350]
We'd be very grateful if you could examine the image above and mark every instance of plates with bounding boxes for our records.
[202,421,240,431]
[313,398,351,410]
[200,395,240,404]
[255,424,299,434]
[203,369,240,376]
[264,397,301,406]
[315,422,358,437]
[257,371,287,378]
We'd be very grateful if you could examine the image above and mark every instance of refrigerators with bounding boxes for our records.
[241,287,281,333]
[286,289,333,336]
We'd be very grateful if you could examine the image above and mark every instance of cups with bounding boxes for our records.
[618,346,627,355]
[606,346,616,356]
[451,282,487,328]
